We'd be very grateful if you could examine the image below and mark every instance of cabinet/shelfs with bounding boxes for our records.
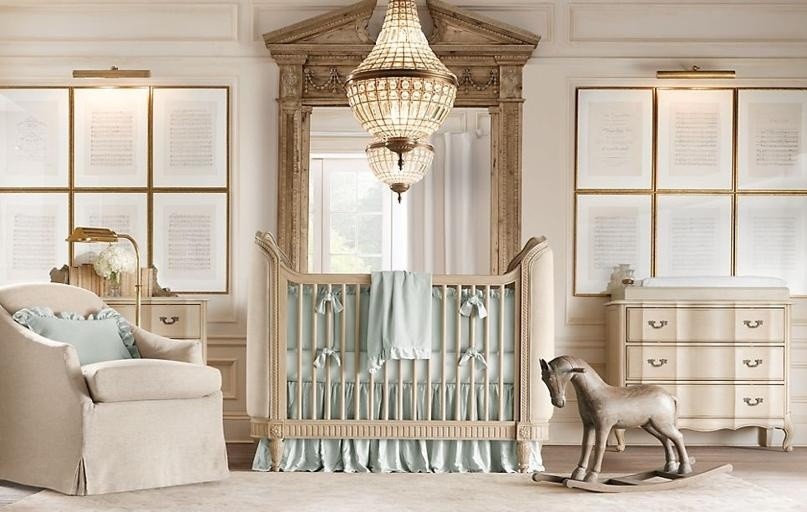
[604,299,795,452]
[103,298,208,367]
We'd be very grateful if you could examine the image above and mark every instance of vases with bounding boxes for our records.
[109,285,118,296]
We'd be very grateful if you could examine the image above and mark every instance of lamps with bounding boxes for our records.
[343,1,461,170]
[366,139,434,205]
[67,228,141,328]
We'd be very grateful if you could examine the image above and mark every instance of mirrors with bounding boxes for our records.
[262,0,540,276]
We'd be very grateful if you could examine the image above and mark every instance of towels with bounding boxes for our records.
[365,270,433,374]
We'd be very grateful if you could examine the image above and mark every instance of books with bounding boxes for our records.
[47,264,153,297]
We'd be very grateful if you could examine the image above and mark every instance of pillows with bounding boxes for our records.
[12,304,140,365]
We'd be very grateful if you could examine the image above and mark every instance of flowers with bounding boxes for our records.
[94,245,135,285]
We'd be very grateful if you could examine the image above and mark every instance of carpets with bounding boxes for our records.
[0,463,807,512]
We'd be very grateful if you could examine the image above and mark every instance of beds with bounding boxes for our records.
[246,231,553,473]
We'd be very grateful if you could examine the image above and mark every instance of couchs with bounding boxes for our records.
[0,281,228,497]
